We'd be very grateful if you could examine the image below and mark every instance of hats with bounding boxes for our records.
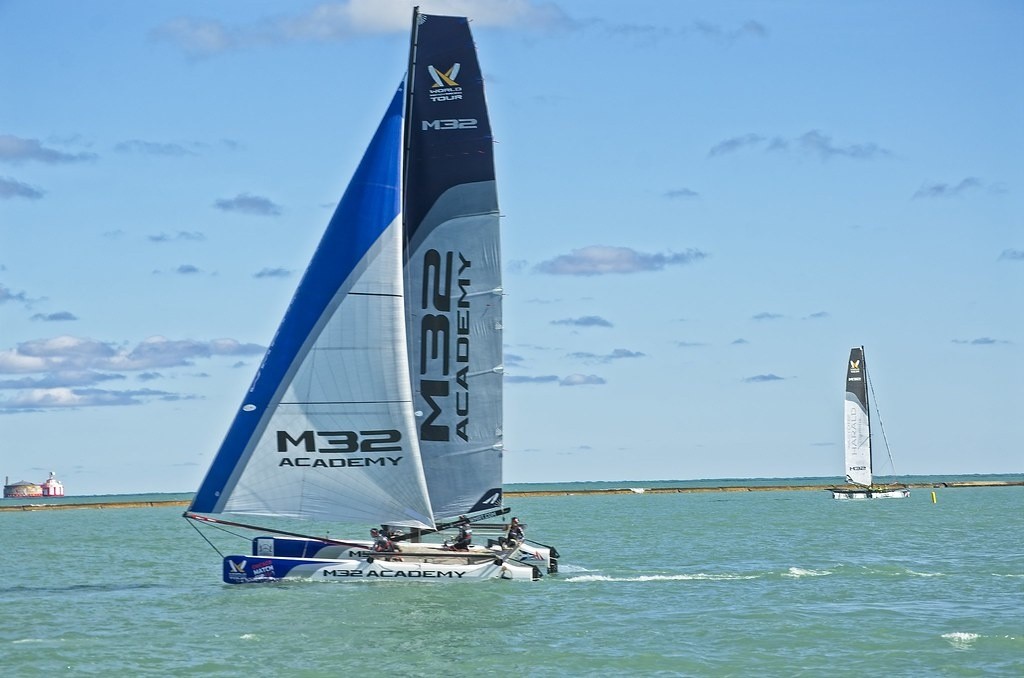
[370,528,379,534]
[459,515,467,519]
[512,519,519,523]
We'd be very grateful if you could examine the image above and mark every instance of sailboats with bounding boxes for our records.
[177,3,561,586]
[823,343,911,501]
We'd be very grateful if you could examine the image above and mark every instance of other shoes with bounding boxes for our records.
[399,557,404,562]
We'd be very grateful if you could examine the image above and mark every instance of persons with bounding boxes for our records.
[448,514,473,551]
[368,525,404,563]
[500,517,525,549]
